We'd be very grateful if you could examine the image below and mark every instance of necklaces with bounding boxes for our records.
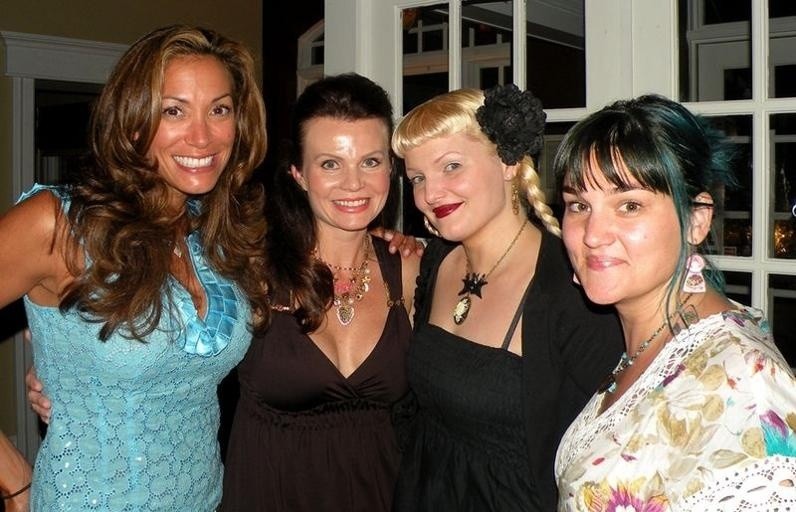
[597,295,692,396]
[312,237,370,325]
[162,237,185,259]
[453,220,529,325]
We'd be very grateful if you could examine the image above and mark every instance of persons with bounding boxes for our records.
[26,73,429,512]
[550,94,796,512]
[0,22,269,512]
[390,87,625,512]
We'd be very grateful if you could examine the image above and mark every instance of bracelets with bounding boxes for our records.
[3,483,31,499]
